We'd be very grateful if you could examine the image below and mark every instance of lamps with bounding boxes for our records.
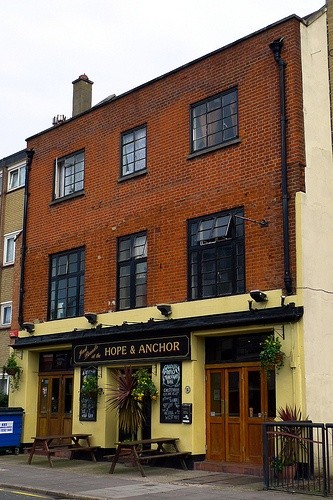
[23,322,34,333]
[157,304,171,316]
[84,312,97,324]
[249,290,269,303]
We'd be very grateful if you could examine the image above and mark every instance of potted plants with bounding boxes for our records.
[267,403,310,485]
[4,355,21,376]
[80,374,99,398]
[131,375,159,405]
[258,334,285,365]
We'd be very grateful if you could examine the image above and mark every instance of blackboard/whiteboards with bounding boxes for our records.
[79,365,97,421]
[160,360,182,424]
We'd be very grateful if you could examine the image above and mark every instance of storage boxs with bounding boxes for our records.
[0,407,26,454]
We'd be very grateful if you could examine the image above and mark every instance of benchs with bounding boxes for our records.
[23,434,101,468]
[104,437,192,477]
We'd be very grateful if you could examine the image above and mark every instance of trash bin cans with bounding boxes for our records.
[0,407,25,455]
